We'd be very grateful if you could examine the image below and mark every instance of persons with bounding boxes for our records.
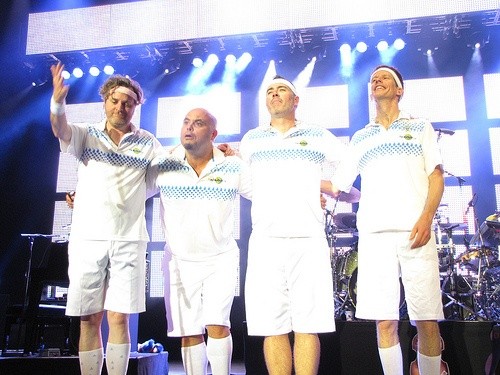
[333,64,445,375]
[235,76,350,375]
[49,60,235,375]
[338,173,361,203]
[65,105,328,375]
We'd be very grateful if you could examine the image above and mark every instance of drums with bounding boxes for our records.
[336,250,407,312]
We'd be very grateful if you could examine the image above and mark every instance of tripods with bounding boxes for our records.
[325,133,500,321]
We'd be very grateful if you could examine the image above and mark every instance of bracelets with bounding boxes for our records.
[50,97,66,114]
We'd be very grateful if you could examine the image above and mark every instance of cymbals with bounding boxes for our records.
[331,212,358,229]
[330,185,360,204]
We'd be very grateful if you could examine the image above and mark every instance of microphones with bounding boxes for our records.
[441,224,459,232]
[436,128,455,135]
